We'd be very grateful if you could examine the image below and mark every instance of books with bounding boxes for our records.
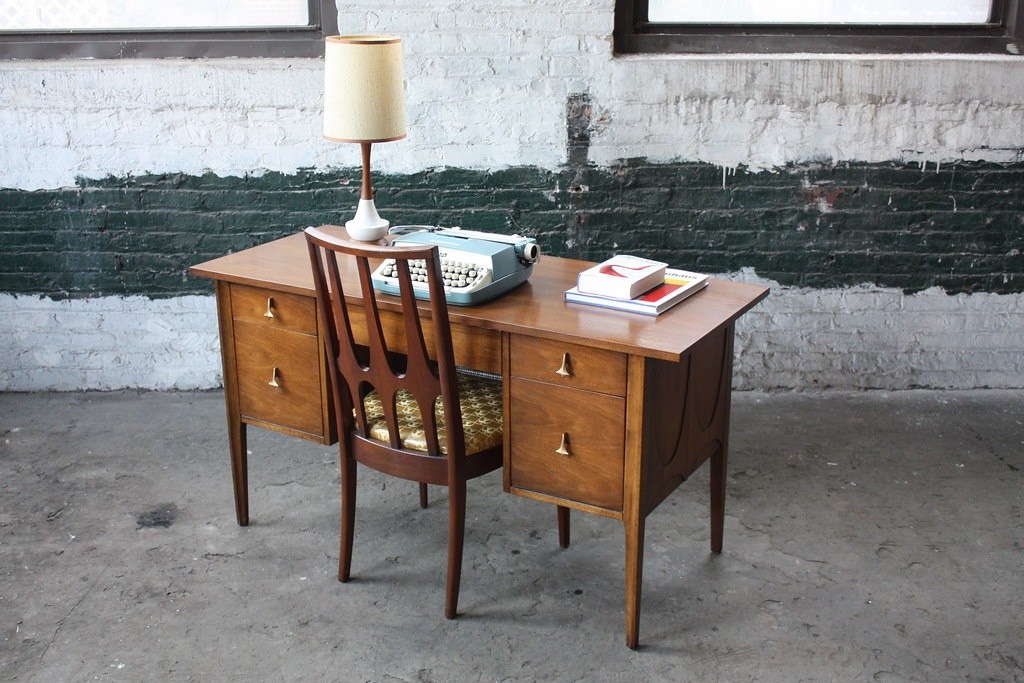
[577,255,669,300]
[563,268,710,317]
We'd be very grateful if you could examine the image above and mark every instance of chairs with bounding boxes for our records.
[303,223,571,620]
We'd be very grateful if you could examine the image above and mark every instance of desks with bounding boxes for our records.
[188,222,772,651]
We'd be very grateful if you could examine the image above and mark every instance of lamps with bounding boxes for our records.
[321,35,406,240]
